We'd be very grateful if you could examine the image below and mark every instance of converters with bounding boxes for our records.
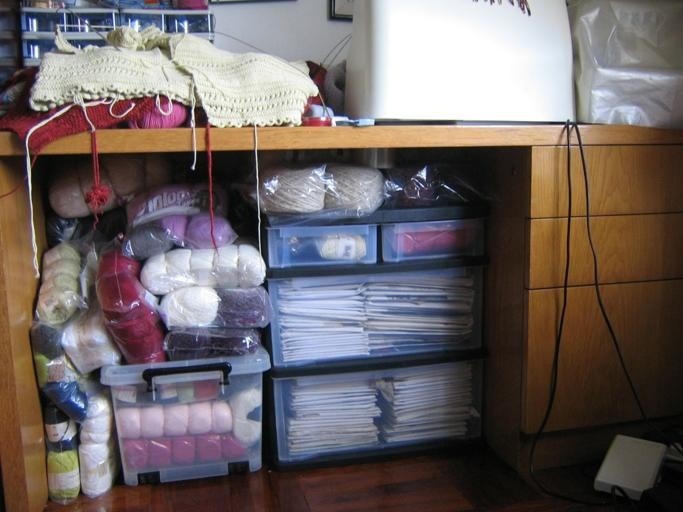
[594,435,667,500]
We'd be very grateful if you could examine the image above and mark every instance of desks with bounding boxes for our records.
[0,124,683,512]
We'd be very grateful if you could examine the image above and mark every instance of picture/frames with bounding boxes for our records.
[330,0,353,21]
